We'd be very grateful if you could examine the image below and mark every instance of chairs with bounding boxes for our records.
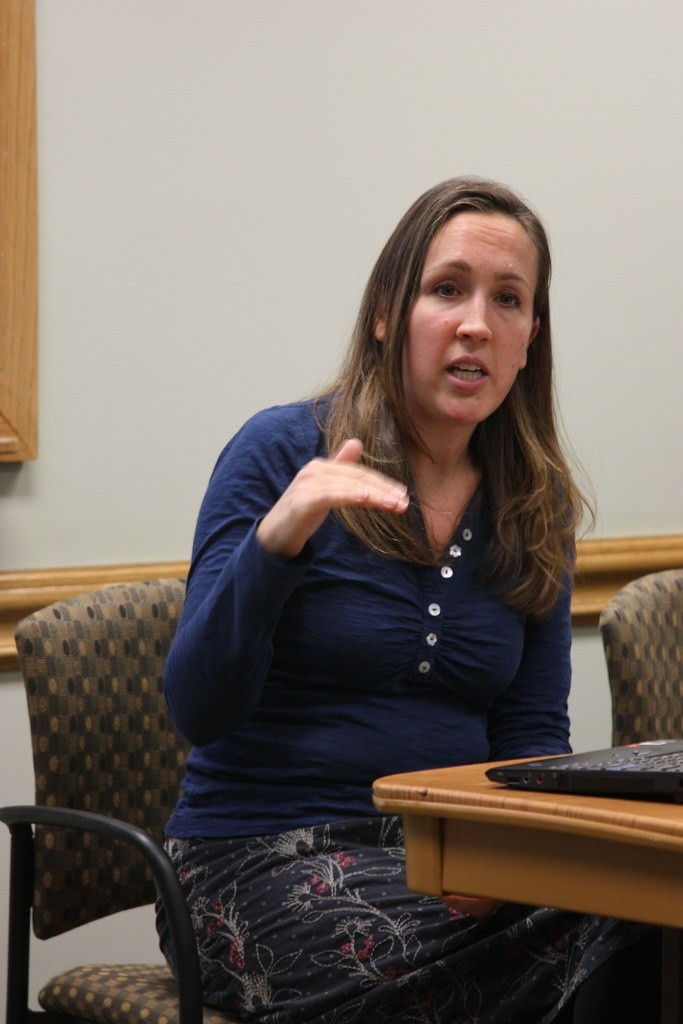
[597,567,683,746]
[0,576,226,1024]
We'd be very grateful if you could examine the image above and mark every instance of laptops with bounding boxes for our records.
[485,738,683,806]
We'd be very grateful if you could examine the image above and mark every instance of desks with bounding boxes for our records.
[371,755,683,1024]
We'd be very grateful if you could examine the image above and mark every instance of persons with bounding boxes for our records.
[156,174,663,1024]
